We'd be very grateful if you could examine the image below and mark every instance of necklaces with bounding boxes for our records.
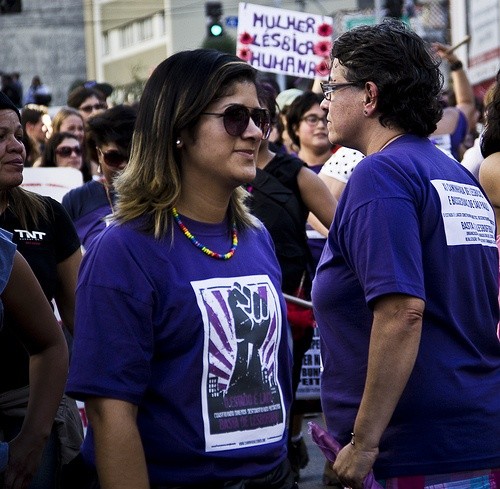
[171,206,238,259]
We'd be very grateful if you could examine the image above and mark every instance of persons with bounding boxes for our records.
[310,18,500,489]
[0,68,142,489]
[238,77,367,344]
[426,40,500,245]
[64,49,311,489]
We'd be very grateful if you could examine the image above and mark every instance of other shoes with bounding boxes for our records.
[293,435,310,470]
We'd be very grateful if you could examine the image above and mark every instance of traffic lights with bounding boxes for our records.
[207,20,223,38]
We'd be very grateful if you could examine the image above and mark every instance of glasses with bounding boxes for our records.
[56,146,82,157]
[98,149,131,167]
[82,104,103,113]
[319,82,358,101]
[201,104,272,140]
[300,114,327,126]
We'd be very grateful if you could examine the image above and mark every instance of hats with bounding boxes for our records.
[274,88,305,114]
[84,81,113,99]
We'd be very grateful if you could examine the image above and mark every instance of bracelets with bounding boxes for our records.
[449,61,462,71]
